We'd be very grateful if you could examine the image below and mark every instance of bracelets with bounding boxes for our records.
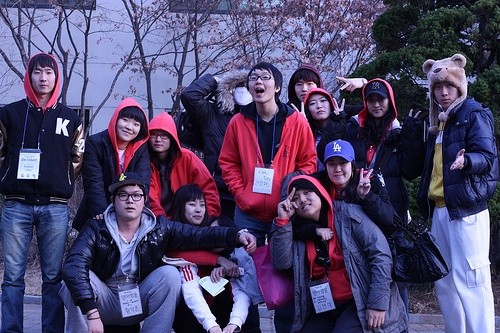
[87,310,100,320]
[362,78,367,83]
[238,228,248,234]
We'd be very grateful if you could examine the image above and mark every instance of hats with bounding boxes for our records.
[109,172,147,194]
[324,139,355,162]
[423,54,467,135]
[365,80,389,98]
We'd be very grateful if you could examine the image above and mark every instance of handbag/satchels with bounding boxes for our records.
[388,220,450,282]
[229,247,264,306]
[252,244,294,310]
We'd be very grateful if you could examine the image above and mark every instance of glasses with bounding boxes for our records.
[149,132,170,139]
[115,192,145,201]
[248,74,274,80]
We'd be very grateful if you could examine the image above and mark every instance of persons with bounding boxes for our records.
[72,97,153,230]
[148,112,222,226]
[269,174,408,333]
[291,76,348,162]
[219,62,318,333]
[181,65,253,222]
[324,138,407,310]
[58,172,257,333]
[0,52,85,333]
[344,79,423,223]
[287,63,368,120]
[161,183,262,333]
[422,54,500,333]
